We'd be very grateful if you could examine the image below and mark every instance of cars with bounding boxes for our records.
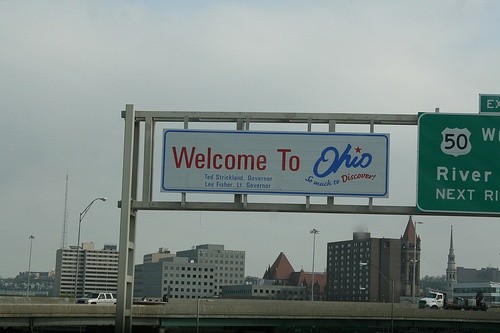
[73,292,117,305]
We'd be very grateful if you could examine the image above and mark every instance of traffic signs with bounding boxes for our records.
[416,112,500,215]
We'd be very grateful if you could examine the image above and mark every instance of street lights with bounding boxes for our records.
[411,221,424,304]
[74,196,108,298]
[196,298,214,333]
[309,229,320,301]
[27,235,37,299]
[359,262,395,333]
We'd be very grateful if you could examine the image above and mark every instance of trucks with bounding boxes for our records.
[418,290,467,310]
